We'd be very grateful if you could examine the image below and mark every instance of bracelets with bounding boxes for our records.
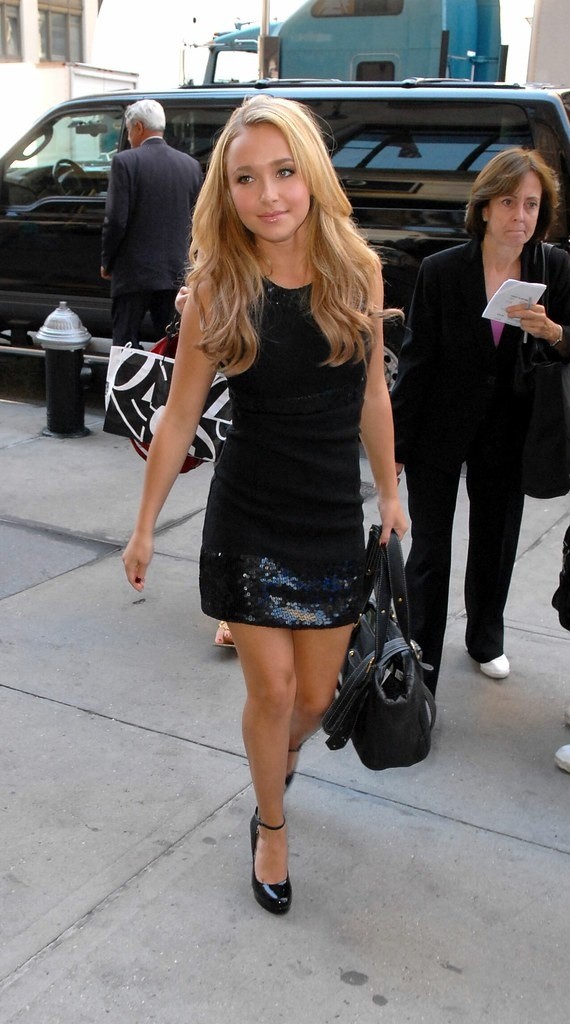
[552,325,562,345]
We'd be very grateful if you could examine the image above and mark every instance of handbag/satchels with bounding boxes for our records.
[513,241,570,498]
[101,311,233,463]
[127,310,209,473]
[321,525,436,770]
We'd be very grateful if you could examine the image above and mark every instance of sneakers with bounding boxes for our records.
[464,640,511,680]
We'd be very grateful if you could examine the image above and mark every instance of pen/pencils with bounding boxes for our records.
[522,295,533,344]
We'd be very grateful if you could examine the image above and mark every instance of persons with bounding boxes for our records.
[100,99,203,357]
[121,95,408,916]
[389,148,570,723]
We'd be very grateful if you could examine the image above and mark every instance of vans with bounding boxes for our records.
[0,74,570,397]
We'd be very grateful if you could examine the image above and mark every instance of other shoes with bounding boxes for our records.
[213,619,235,647]
[554,744,570,773]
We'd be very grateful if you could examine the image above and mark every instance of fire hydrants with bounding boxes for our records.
[28,300,92,439]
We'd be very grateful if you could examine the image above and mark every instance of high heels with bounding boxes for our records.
[283,744,303,795]
[249,806,292,916]
